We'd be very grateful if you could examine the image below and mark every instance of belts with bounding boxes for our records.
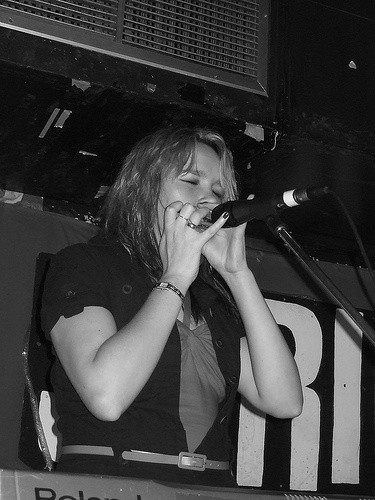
[59,444,231,472]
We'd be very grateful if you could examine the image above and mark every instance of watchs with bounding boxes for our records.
[151,282,186,312]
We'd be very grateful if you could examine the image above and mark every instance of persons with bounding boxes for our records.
[31,124,303,485]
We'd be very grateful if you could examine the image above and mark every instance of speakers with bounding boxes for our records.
[0,0,269,126]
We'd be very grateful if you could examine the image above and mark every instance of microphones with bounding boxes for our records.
[212,185,329,228]
